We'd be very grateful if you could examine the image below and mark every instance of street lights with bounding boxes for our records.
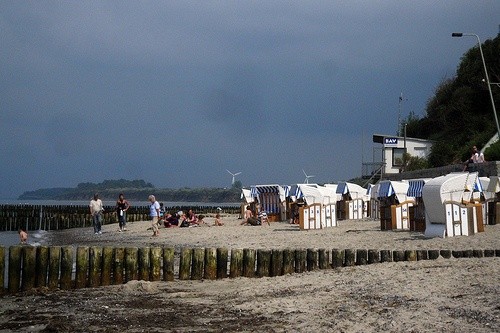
[452,33,499,138]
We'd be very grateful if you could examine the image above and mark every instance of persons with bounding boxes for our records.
[89,193,103,235]
[463,145,484,163]
[17,230,28,243]
[241,206,271,226]
[158,202,207,228]
[115,193,130,232]
[149,195,160,236]
[215,209,223,226]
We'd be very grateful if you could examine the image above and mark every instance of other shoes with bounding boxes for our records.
[151,231,161,237]
[95,230,102,235]
[118,227,127,232]
